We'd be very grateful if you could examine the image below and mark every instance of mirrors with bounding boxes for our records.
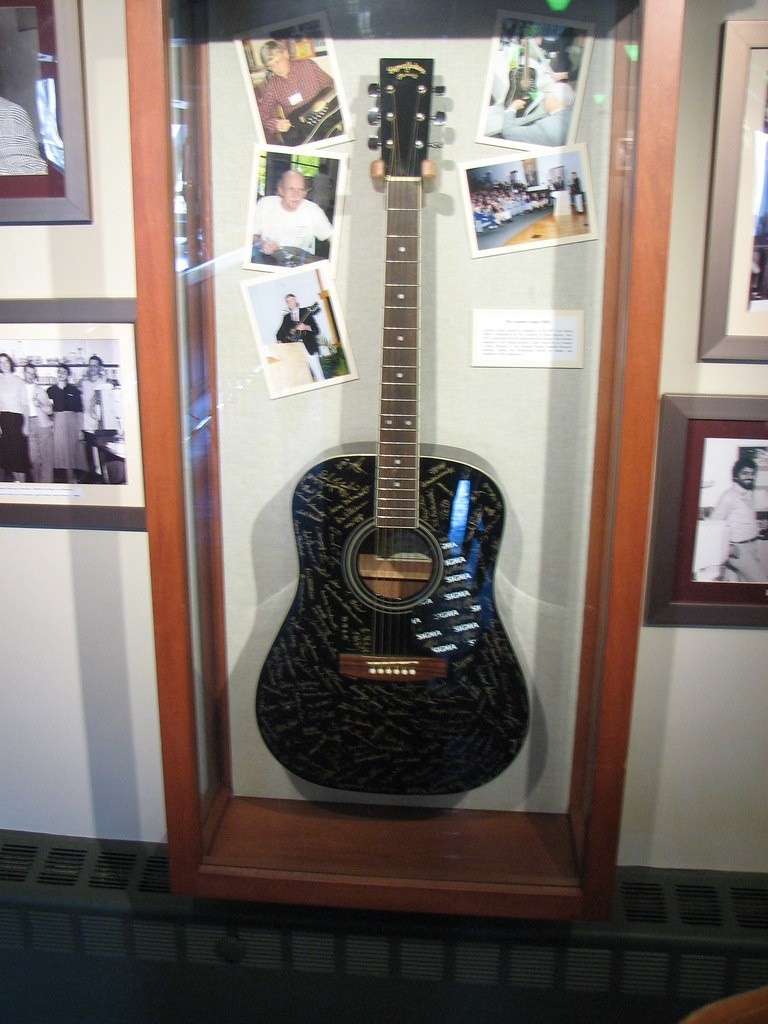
[0,0,93,227]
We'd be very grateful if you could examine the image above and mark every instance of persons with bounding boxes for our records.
[260,40,336,140]
[471,172,583,230]
[0,353,117,484]
[254,163,333,256]
[707,458,768,582]
[276,293,325,382]
[485,22,584,145]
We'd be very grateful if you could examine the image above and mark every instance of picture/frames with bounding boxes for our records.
[641,392,768,629]
[696,19,768,364]
[0,296,147,532]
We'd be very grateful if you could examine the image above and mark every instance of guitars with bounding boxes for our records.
[281,302,320,343]
[280,86,342,147]
[503,31,538,128]
[252,57,530,796]
